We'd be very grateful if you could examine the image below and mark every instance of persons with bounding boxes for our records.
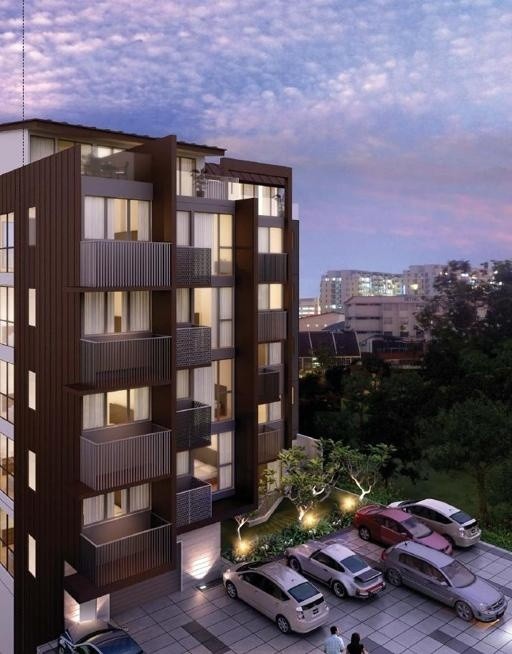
[322,625,346,654]
[342,632,368,654]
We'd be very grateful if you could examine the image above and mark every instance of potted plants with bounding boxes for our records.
[190,168,210,198]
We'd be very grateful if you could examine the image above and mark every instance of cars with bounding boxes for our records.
[356,485,510,625]
[283,533,386,603]
[55,617,147,654]
[217,556,331,637]
